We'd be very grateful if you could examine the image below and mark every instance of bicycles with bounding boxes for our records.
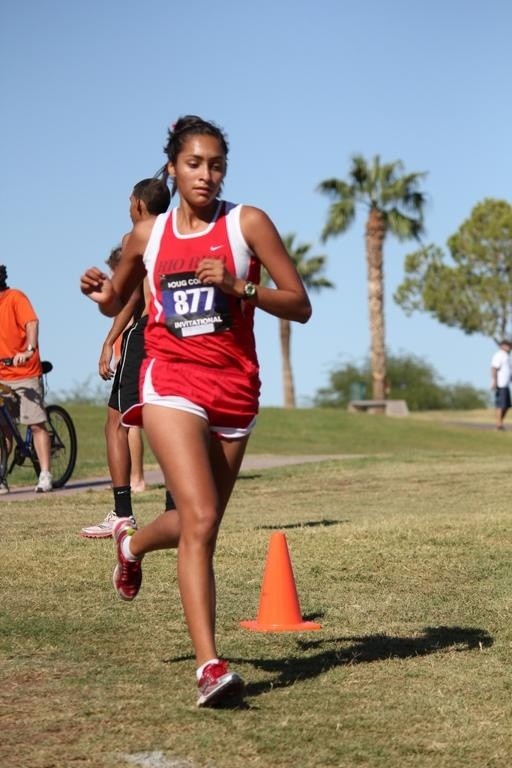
[0,358,77,489]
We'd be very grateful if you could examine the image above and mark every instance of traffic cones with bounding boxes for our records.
[240,531,321,632]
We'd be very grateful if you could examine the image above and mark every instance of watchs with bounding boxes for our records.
[241,279,257,301]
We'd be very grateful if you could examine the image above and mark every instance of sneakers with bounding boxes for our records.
[35,471,53,493]
[196,659,241,707]
[112,517,142,601]
[81,510,137,538]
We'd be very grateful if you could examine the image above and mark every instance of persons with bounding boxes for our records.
[80,114,312,711]
[105,244,148,496]
[490,339,511,432]
[0,263,56,493]
[81,176,175,542]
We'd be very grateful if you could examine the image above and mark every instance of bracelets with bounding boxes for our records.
[26,344,36,353]
